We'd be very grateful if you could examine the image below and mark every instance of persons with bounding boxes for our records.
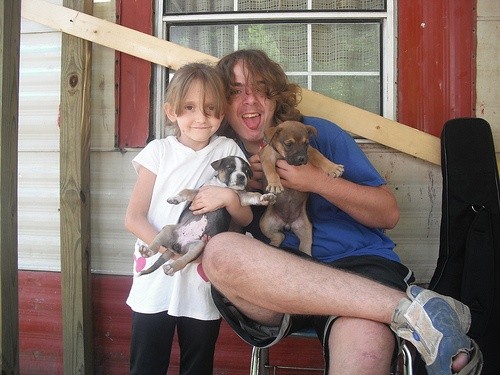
[202,49,484,375]
[125,63,253,375]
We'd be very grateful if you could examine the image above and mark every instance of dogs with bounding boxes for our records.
[139,156,277,276]
[259,120,344,257]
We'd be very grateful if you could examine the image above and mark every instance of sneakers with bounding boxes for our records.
[388,284,484,375]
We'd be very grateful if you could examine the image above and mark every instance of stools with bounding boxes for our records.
[247,331,417,375]
